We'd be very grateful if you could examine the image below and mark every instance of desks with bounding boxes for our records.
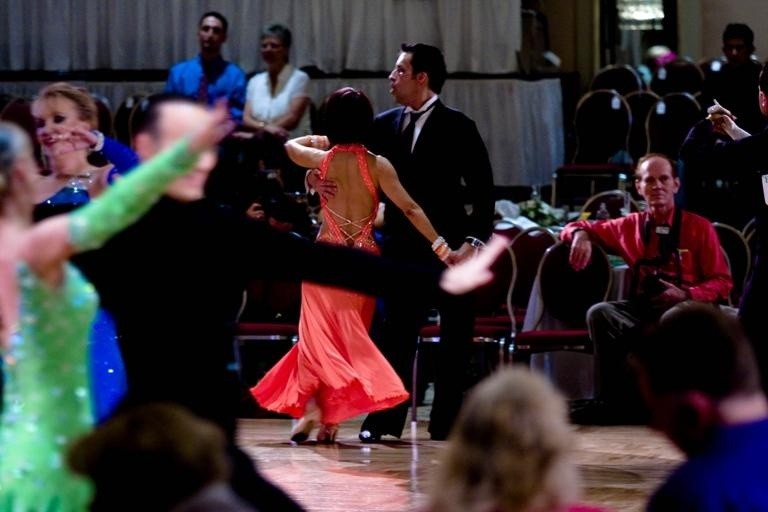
[0,70,565,206]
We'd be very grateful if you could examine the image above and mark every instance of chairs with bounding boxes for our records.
[234,275,299,367]
[589,63,642,99]
[506,226,556,368]
[621,90,659,168]
[113,90,150,148]
[412,248,519,426]
[493,220,524,236]
[129,91,202,153]
[646,91,700,169]
[1,94,34,137]
[507,234,615,418]
[82,90,111,140]
[706,223,749,313]
[558,90,634,205]
[651,59,704,97]
[580,189,640,230]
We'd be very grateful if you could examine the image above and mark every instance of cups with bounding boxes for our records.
[519,199,539,222]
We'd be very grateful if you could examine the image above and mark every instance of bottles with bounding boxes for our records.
[596,201,610,219]
[531,184,542,204]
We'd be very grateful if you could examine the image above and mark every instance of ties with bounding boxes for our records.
[396,112,423,152]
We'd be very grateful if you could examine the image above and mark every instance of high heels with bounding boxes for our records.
[291,416,319,443]
[317,423,338,443]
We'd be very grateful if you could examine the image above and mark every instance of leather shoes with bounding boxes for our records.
[358,430,374,440]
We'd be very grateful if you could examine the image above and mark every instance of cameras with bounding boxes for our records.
[641,272,672,299]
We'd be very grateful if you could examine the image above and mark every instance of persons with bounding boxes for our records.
[559,151,734,427]
[414,361,617,512]
[684,22,768,214]
[641,300,768,511]
[2,10,496,512]
